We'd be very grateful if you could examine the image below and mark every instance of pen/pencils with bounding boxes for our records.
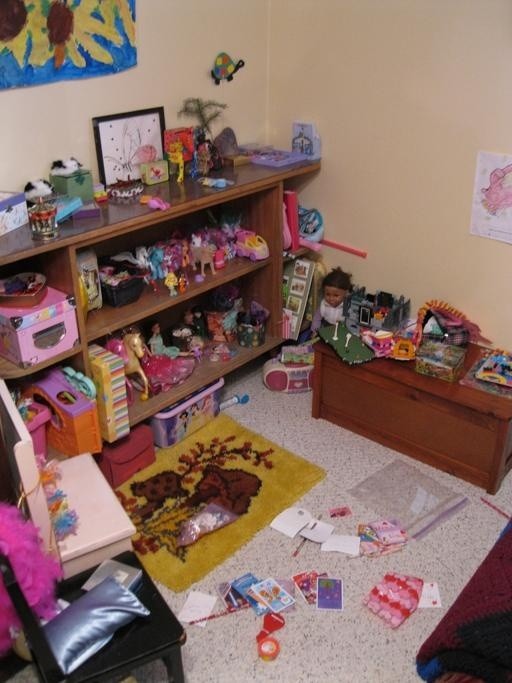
[294,536,306,556]
[189,607,236,625]
[229,591,240,609]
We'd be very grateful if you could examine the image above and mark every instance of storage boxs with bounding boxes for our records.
[88,343,131,443]
[150,376,225,448]
[0,286,81,370]
[93,425,156,489]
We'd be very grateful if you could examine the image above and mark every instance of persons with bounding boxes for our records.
[165,273,179,297]
[319,271,351,328]
[188,339,204,363]
[123,326,195,394]
[146,320,193,357]
[178,271,186,292]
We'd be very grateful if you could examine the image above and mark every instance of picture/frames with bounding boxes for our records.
[92,107,166,193]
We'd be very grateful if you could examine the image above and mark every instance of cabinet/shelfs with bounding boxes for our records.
[0,161,321,465]
[312,338,512,496]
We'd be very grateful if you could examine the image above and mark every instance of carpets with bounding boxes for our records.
[114,410,329,593]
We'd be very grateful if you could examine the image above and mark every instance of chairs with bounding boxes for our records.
[0,378,137,581]
[0,550,187,683]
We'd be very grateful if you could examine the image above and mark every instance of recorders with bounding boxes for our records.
[262,351,316,393]
